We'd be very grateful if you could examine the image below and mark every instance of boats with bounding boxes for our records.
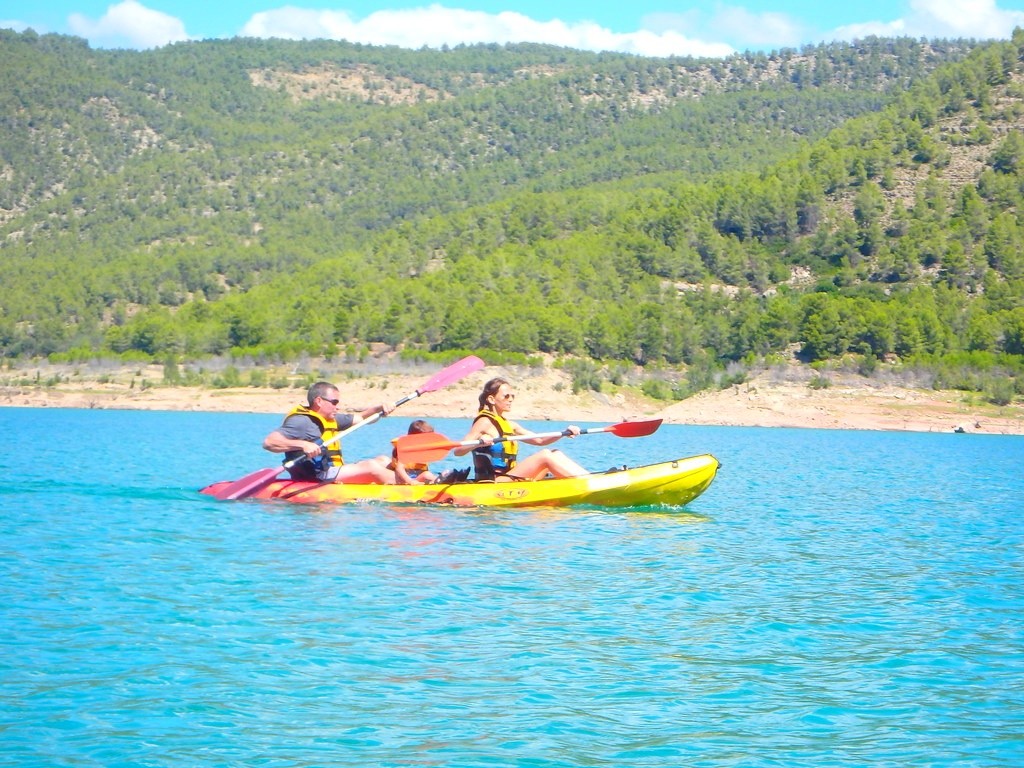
[199,454,724,511]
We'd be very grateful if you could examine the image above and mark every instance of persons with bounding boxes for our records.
[453,377,590,483]
[262,382,403,486]
[392,420,471,486]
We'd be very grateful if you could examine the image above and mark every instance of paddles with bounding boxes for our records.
[216,354,485,500]
[397,418,664,465]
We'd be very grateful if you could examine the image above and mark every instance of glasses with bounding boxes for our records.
[491,393,515,400]
[314,396,340,406]
